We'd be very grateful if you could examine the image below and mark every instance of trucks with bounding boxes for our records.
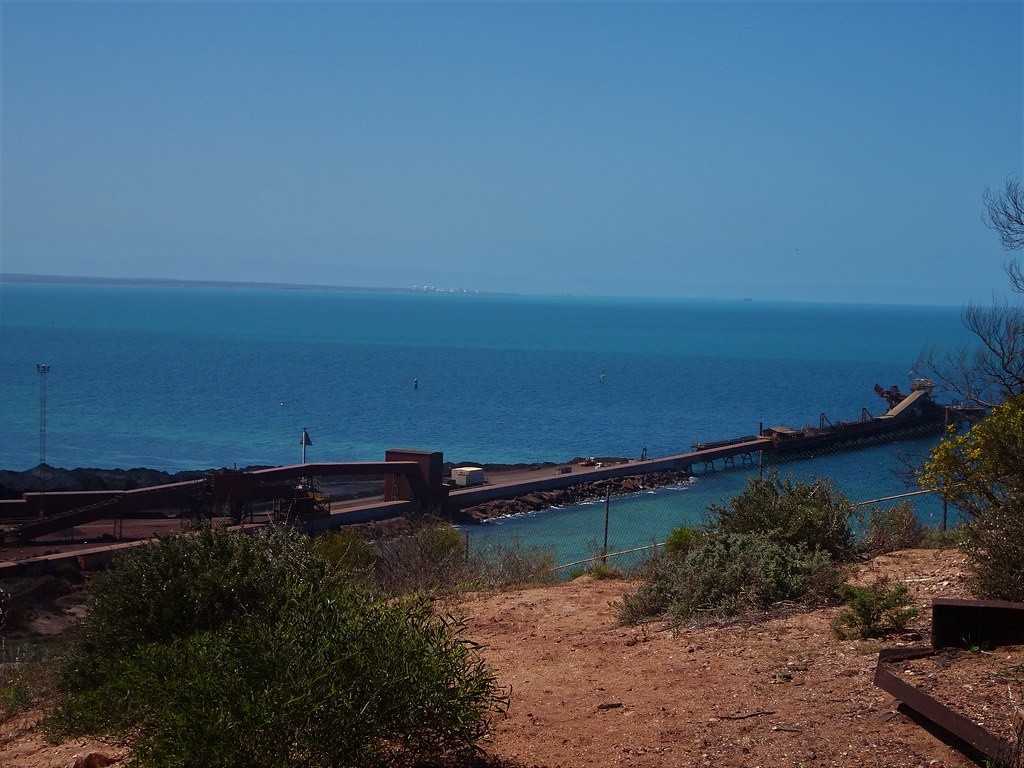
[578,457,596,467]
[594,461,621,470]
[553,466,571,475]
[443,467,483,489]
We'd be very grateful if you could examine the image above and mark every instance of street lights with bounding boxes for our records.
[37,363,52,490]
